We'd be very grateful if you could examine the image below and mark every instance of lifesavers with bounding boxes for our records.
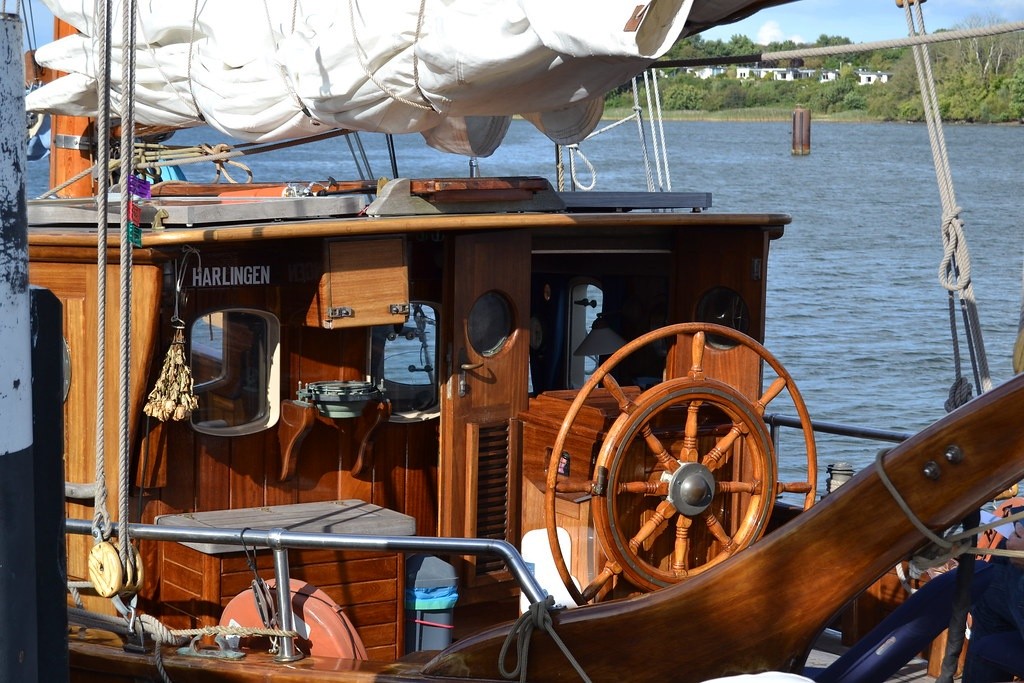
[218,576,366,659]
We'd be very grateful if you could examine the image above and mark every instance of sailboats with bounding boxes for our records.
[2,0,1023,683]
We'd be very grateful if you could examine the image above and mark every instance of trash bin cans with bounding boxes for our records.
[403,552,459,652]
